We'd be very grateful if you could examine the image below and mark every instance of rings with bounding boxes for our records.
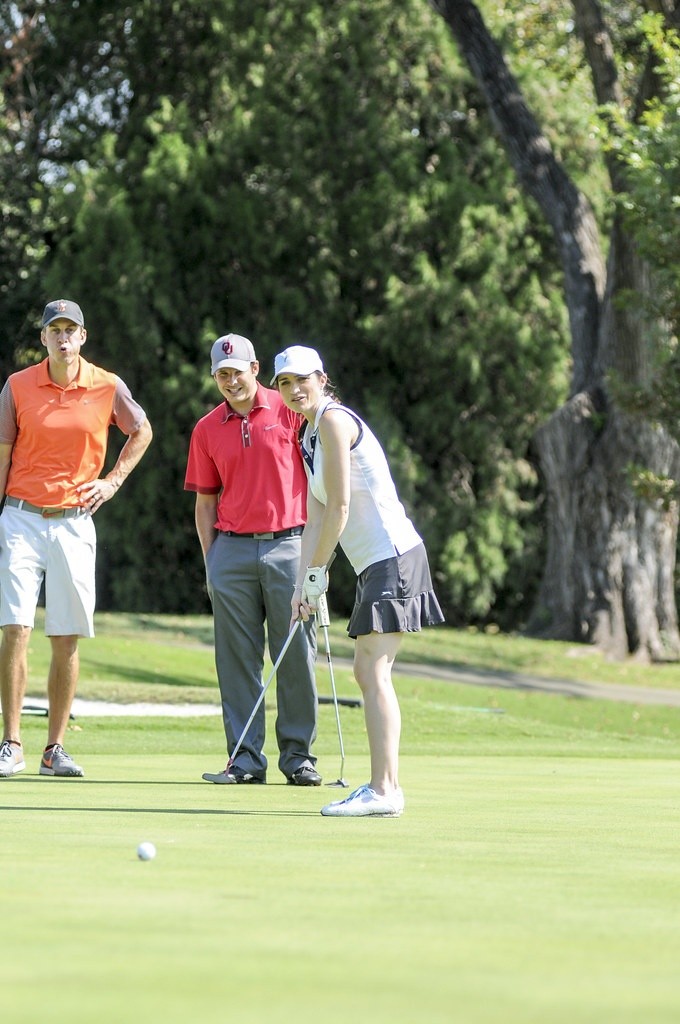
[93,496,98,501]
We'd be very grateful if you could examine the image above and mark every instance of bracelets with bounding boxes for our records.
[294,584,303,591]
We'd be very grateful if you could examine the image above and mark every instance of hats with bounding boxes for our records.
[42,299,84,329]
[269,345,325,387]
[210,333,256,372]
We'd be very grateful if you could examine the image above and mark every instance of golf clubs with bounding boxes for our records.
[202,550,338,784]
[316,590,350,787]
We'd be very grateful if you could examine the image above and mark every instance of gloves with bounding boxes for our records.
[300,565,329,610]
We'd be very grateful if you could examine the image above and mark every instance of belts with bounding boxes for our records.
[4,495,87,519]
[220,526,304,540]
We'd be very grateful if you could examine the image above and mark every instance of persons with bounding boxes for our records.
[184,333,323,785]
[0,299,153,777]
[269,344,445,818]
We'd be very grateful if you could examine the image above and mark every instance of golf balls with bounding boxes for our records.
[137,842,156,861]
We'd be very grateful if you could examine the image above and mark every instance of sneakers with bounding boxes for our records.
[0,741,25,777]
[39,745,84,777]
[321,783,404,819]
[286,767,322,786]
[201,764,266,784]
